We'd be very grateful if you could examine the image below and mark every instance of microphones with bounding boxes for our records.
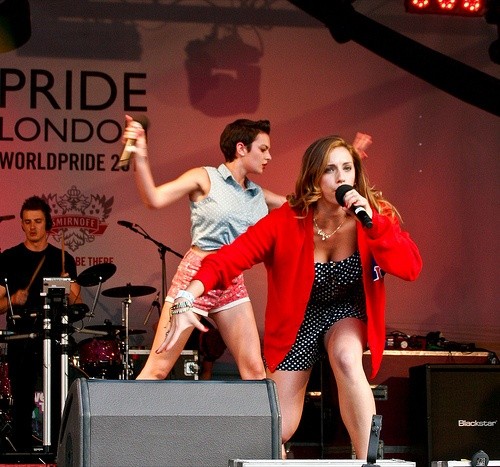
[118,220,139,227]
[144,291,160,326]
[335,185,373,229]
[116,114,149,168]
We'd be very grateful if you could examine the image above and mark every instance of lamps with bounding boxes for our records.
[404,0,489,18]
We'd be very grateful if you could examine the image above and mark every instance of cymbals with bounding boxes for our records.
[67,303,90,323]
[108,329,147,337]
[101,285,156,298]
[86,325,127,332]
[76,262,117,287]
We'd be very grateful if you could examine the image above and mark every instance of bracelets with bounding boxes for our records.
[169,309,190,314]
[174,290,195,307]
[169,301,189,311]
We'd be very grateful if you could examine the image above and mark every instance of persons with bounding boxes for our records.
[156,135,422,460]
[124,112,373,380]
[0,195,80,465]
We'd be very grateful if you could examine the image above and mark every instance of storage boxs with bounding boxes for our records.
[321,350,489,458]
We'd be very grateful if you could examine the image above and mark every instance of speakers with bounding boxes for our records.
[410,363,500,467]
[57,378,282,467]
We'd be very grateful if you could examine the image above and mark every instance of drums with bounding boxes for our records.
[78,336,134,379]
[0,362,12,407]
[13,361,89,442]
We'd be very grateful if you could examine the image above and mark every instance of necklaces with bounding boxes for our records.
[313,216,345,241]
